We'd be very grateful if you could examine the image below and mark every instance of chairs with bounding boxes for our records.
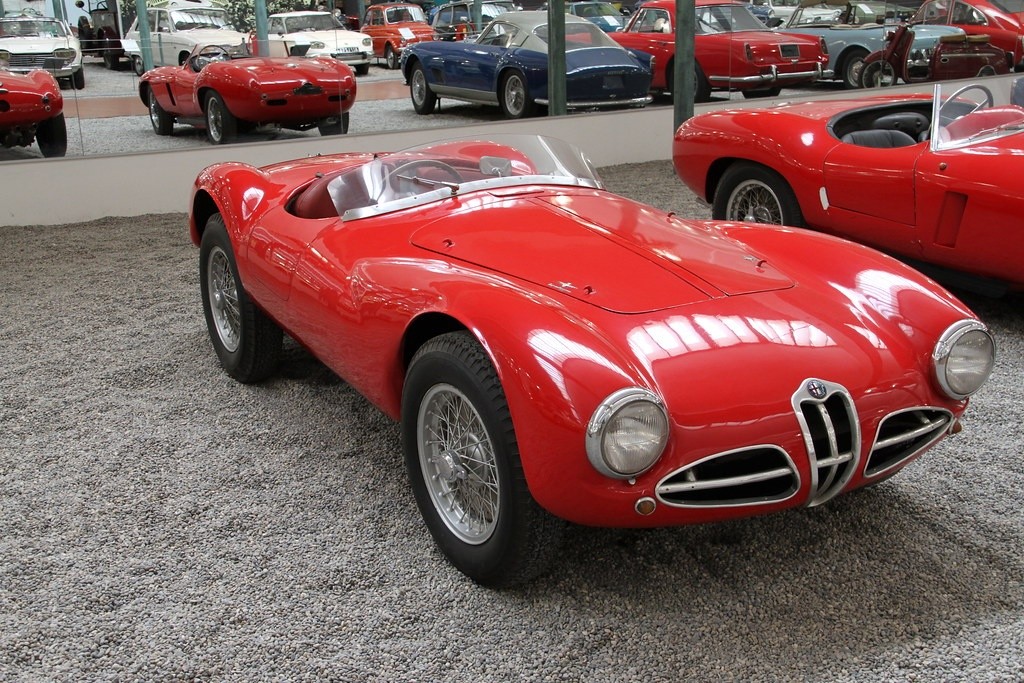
[934,17,987,26]
[495,34,594,48]
[294,163,513,219]
[830,22,880,30]
[840,112,970,148]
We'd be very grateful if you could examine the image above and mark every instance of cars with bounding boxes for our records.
[400,9,654,121]
[771,0,968,86]
[905,0,1023,71]
[359,3,465,70]
[671,81,1022,288]
[259,10,372,76]
[138,45,357,142]
[826,2,916,25]
[561,1,829,97]
[747,0,840,27]
[430,1,517,44]
[78,1,139,68]
[1,16,87,89]
[637,0,775,31]
[0,64,68,160]
[536,1,646,40]
[185,131,996,578]
[122,2,255,75]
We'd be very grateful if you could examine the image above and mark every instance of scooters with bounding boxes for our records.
[858,10,1014,89]
